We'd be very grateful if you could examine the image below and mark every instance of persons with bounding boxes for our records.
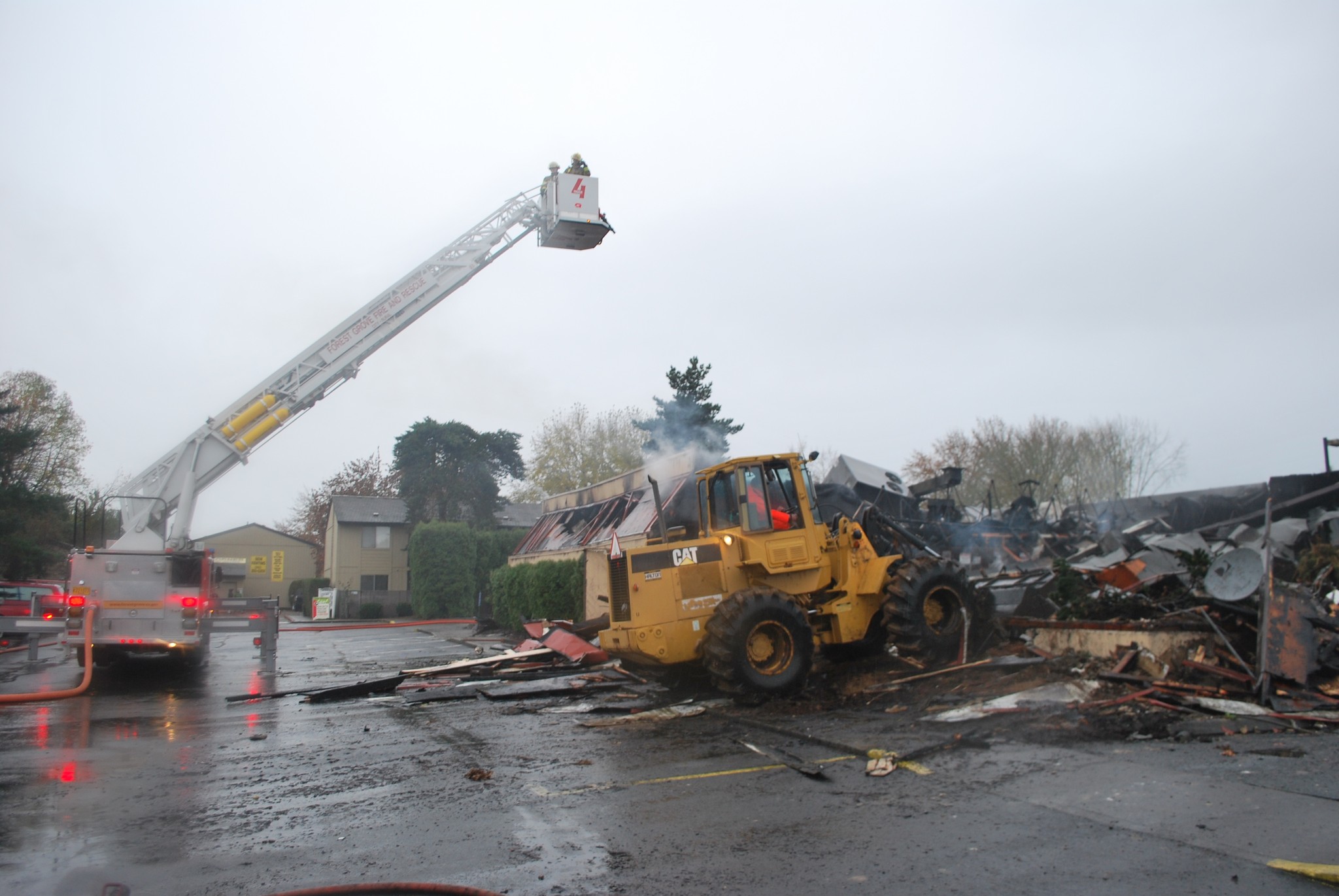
[750,477,798,531]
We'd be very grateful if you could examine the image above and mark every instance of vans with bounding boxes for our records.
[0,580,69,637]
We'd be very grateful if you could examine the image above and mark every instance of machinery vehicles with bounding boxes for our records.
[600,451,978,706]
[56,173,614,689]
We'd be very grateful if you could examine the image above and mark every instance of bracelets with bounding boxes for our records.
[790,514,793,521]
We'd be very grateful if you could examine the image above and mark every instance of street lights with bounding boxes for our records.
[1322,436,1339,472]
